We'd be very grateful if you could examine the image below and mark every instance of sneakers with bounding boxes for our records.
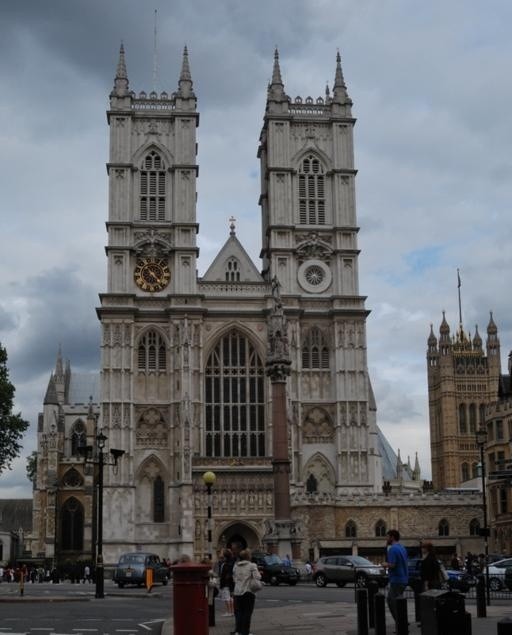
[222,612,234,617]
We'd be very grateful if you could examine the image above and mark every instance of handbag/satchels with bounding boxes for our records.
[437,559,449,583]
[249,579,263,591]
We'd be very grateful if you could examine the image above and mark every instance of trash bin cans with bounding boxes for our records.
[418,589,466,634]
[169,562,212,635]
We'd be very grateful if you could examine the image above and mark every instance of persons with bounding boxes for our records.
[158,552,320,585]
[381,529,411,635]
[217,548,236,618]
[230,549,263,635]
[198,558,220,627]
[449,550,487,576]
[416,539,441,628]
[0,563,95,585]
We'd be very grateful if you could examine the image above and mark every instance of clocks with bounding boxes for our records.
[134,256,171,291]
